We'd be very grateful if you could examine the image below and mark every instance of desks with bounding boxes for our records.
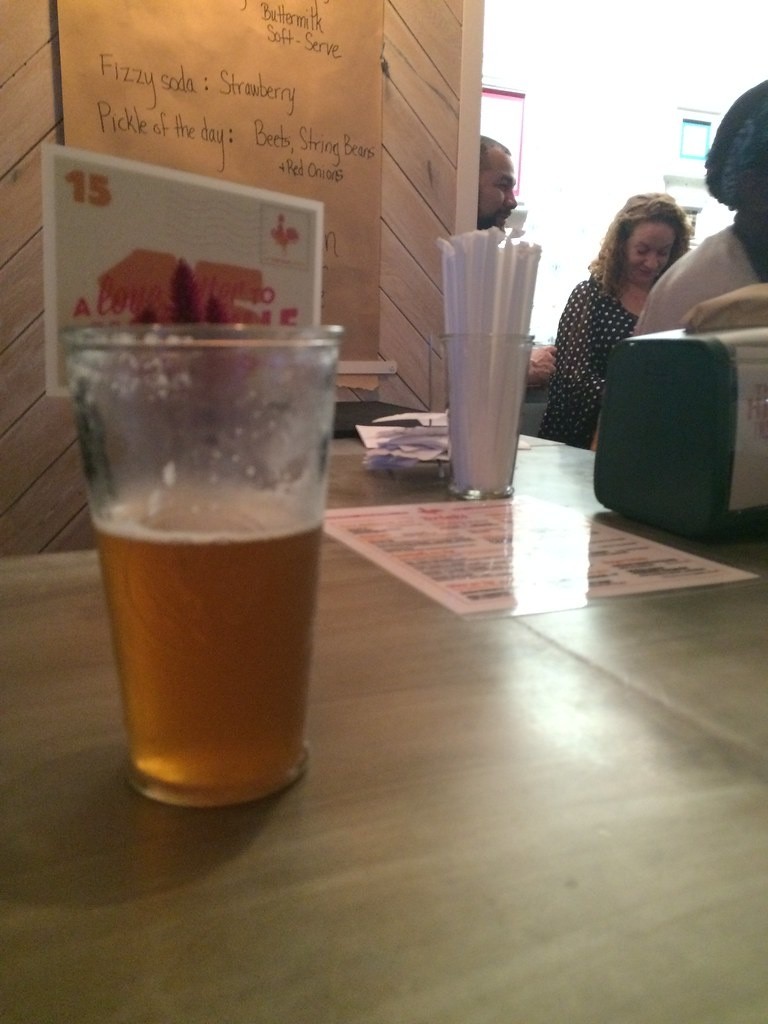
[0,434,768,1024]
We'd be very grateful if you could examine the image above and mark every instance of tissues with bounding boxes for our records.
[592,280,768,547]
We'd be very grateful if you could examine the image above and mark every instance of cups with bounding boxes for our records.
[440,332,535,501]
[57,322,347,808]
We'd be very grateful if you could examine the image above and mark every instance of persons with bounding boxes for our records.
[633,81,768,336]
[538,193,690,449]
[476,135,557,386]
